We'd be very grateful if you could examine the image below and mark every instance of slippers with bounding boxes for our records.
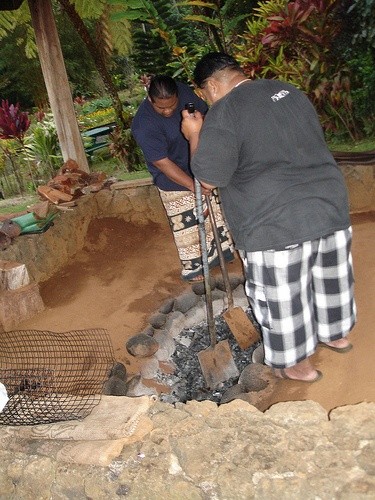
[317,341,353,352]
[271,364,323,382]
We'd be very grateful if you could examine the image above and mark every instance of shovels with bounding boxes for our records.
[186,104,264,390]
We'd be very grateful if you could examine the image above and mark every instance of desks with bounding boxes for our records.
[80,125,116,145]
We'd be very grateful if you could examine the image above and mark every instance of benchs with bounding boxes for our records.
[85,141,111,162]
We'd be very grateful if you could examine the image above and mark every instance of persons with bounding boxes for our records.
[131,75,238,284]
[181,53,358,382]
